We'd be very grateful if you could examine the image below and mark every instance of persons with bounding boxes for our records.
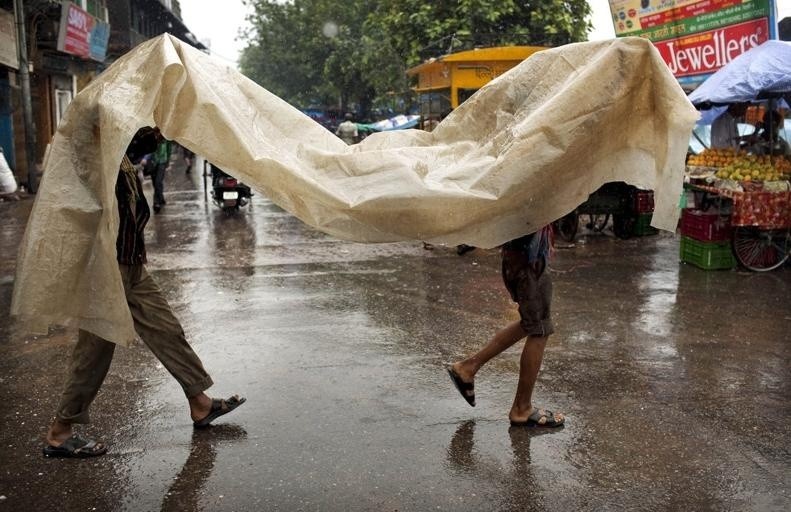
[335,111,359,146]
[709,100,759,153]
[443,219,570,433]
[41,105,250,461]
[0,144,18,206]
[753,110,791,161]
[182,146,195,176]
[143,141,171,213]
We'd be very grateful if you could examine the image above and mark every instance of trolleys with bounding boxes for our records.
[553,180,630,242]
[685,183,789,272]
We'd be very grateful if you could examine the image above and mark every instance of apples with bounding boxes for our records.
[686,148,791,181]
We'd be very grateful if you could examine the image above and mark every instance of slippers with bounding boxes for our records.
[510,407,564,429]
[42,434,106,459]
[446,366,475,407]
[192,396,246,427]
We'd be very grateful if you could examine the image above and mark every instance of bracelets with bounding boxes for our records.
[742,135,747,141]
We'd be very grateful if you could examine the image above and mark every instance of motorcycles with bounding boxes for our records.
[208,163,253,216]
